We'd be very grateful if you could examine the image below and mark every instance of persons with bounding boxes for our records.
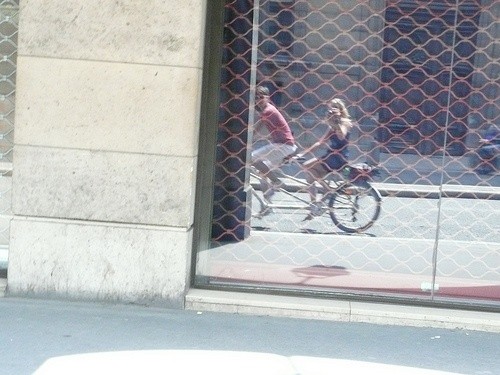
[288,97,354,220]
[478,136,500,176]
[250,85,299,219]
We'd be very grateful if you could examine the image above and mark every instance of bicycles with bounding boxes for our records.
[249,154,383,233]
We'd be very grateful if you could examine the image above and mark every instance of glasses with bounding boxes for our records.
[255,95,270,99]
